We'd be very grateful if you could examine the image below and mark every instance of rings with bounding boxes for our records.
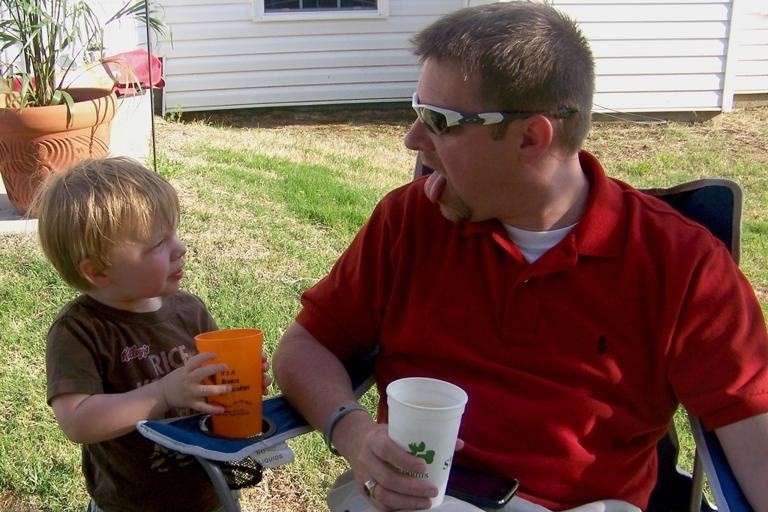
[364,479,376,498]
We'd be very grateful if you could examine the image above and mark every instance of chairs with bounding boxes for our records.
[632,176,756,511]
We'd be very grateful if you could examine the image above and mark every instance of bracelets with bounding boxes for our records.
[322,403,371,456]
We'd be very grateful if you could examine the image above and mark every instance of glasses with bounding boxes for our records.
[410,90,581,138]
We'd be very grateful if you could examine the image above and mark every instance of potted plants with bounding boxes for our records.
[0,1,176,220]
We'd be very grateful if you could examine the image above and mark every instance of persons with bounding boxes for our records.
[272,0,768,512]
[35,159,272,512]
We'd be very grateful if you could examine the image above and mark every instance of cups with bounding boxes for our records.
[386,376,467,509]
[193,330,264,439]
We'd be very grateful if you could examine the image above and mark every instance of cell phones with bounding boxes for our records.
[444,463,518,508]
